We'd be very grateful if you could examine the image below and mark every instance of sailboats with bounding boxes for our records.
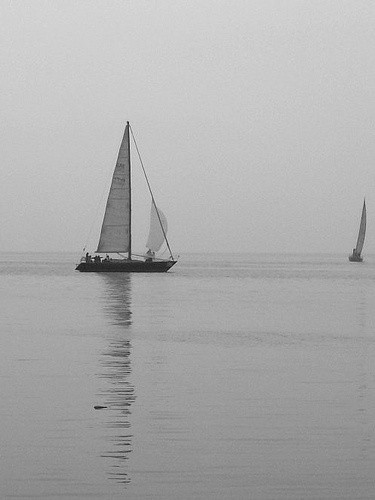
[348,196,367,262]
[73,120,178,273]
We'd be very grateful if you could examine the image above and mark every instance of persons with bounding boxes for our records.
[85,252,109,264]
